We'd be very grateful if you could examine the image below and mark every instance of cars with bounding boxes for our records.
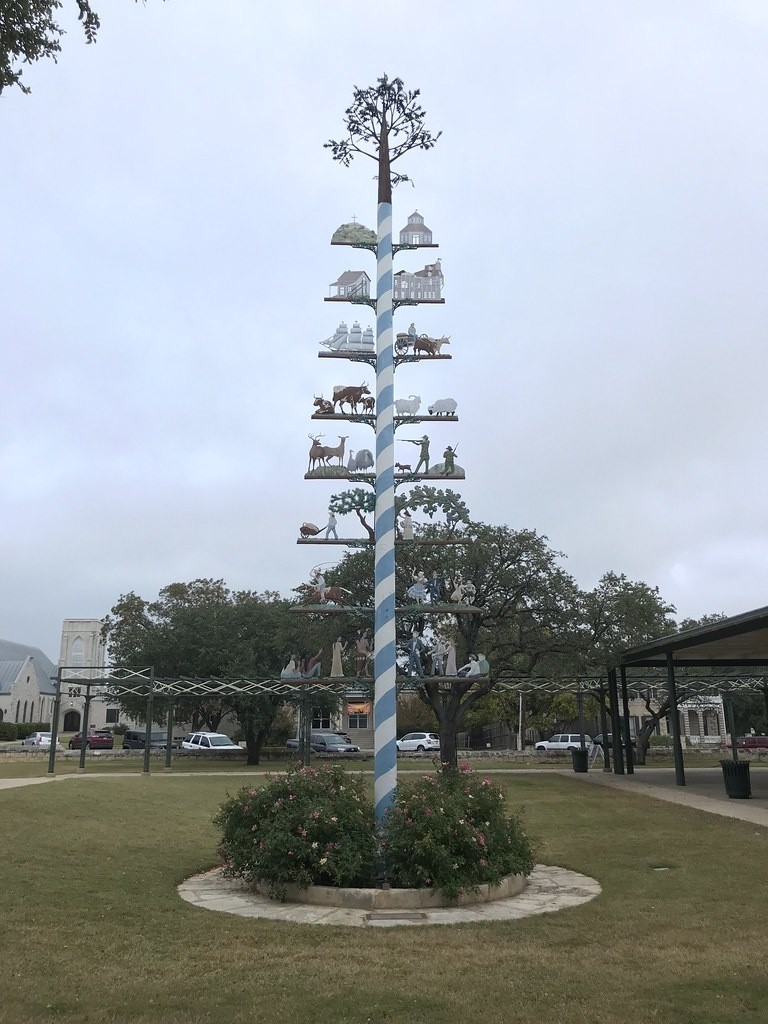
[396,732,440,751]
[21,731,59,746]
[592,733,638,748]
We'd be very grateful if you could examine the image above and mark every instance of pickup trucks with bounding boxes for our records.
[286,733,359,753]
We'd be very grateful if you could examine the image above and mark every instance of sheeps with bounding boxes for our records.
[393,395,457,417]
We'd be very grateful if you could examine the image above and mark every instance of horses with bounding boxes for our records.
[296,583,353,609]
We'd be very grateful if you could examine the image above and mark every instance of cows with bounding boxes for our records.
[413,334,451,355]
[313,381,375,416]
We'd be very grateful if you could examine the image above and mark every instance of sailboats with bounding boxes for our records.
[319,321,376,356]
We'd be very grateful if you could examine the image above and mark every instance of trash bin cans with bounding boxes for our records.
[570,747,589,772]
[718,759,752,799]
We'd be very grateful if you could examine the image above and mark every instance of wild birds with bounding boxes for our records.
[347,449,374,473]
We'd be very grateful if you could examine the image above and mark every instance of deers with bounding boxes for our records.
[308,433,349,472]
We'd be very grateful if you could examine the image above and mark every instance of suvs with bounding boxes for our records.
[333,732,351,744]
[69,728,115,750]
[123,729,178,750]
[725,736,768,749]
[182,731,243,750]
[534,733,591,750]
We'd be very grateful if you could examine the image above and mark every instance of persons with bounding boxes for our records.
[311,568,325,603]
[411,435,430,475]
[408,567,428,605]
[408,323,418,339]
[408,631,425,677]
[331,636,348,677]
[356,630,373,677]
[281,653,301,679]
[440,446,457,475]
[427,637,490,677]
[323,512,338,540]
[451,576,477,607]
[400,510,414,540]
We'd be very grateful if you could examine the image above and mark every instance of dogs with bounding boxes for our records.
[395,462,412,473]
